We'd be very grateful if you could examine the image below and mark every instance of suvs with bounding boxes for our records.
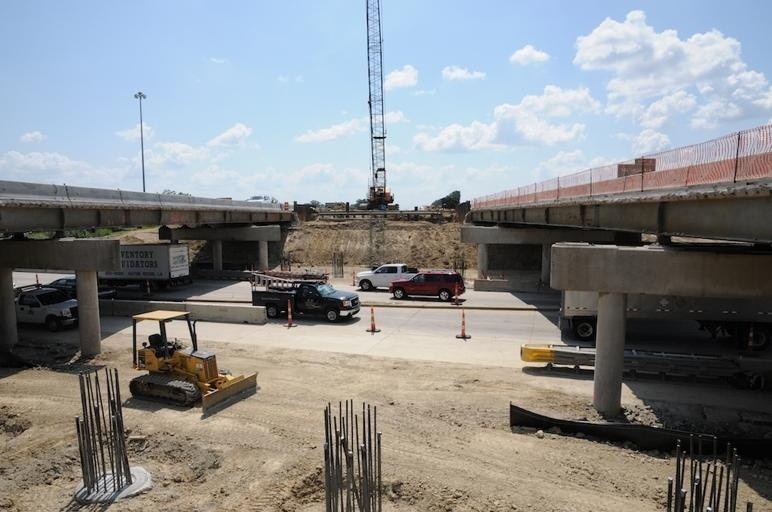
[388,270,465,302]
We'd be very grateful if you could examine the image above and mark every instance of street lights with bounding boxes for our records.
[133,91,148,191]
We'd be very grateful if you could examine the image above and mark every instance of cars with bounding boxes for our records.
[246,195,274,204]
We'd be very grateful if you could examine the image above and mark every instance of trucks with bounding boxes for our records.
[558,289,771,354]
[97,244,192,293]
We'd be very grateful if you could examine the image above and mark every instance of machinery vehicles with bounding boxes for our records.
[127,307,259,418]
[360,0,400,212]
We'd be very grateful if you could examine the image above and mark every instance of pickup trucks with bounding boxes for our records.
[13,277,119,331]
[356,263,419,290]
[247,264,361,323]
